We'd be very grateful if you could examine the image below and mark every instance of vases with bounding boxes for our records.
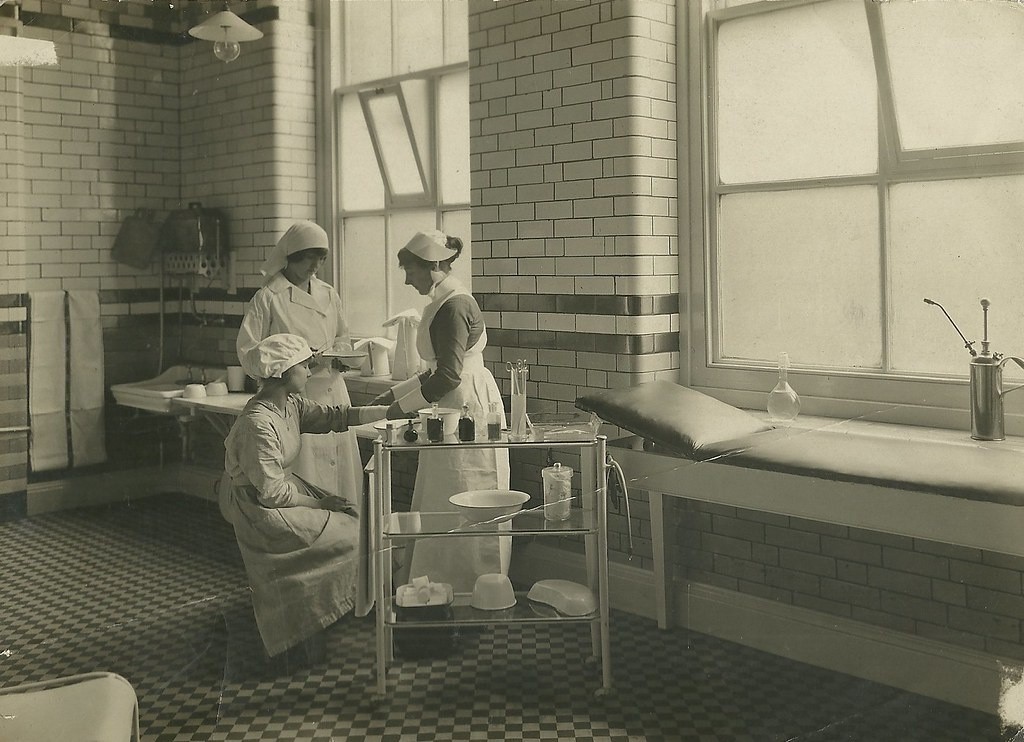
[391,316,420,380]
[359,341,390,376]
[766,353,802,421]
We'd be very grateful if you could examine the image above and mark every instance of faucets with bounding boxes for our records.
[201,368,206,381]
[187,366,193,380]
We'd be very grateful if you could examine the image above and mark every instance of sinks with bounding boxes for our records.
[110,383,190,412]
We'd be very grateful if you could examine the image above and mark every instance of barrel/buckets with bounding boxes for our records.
[112,202,228,269]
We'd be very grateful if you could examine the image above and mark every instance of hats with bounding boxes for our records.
[242,333,314,380]
[404,229,458,262]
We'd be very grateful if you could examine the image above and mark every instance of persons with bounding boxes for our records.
[236,221,365,513]
[369,229,513,637]
[216,332,417,659]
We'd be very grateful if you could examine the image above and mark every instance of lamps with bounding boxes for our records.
[187,0,263,63]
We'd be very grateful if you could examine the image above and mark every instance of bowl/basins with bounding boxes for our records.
[469,573,517,610]
[183,382,230,398]
[418,408,460,435]
[448,489,532,524]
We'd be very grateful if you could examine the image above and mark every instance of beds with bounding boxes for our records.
[573,379,1024,662]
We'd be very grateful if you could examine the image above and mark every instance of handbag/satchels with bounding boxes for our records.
[108,206,160,267]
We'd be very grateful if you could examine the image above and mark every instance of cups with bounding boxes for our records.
[226,366,246,392]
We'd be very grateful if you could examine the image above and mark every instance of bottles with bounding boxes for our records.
[403,419,418,442]
[486,401,502,441]
[427,402,445,443]
[766,352,802,422]
[459,404,475,442]
[541,462,573,520]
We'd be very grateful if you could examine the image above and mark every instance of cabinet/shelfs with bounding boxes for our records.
[365,421,620,707]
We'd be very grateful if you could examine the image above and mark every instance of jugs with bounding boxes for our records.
[361,338,391,376]
[391,312,421,380]
